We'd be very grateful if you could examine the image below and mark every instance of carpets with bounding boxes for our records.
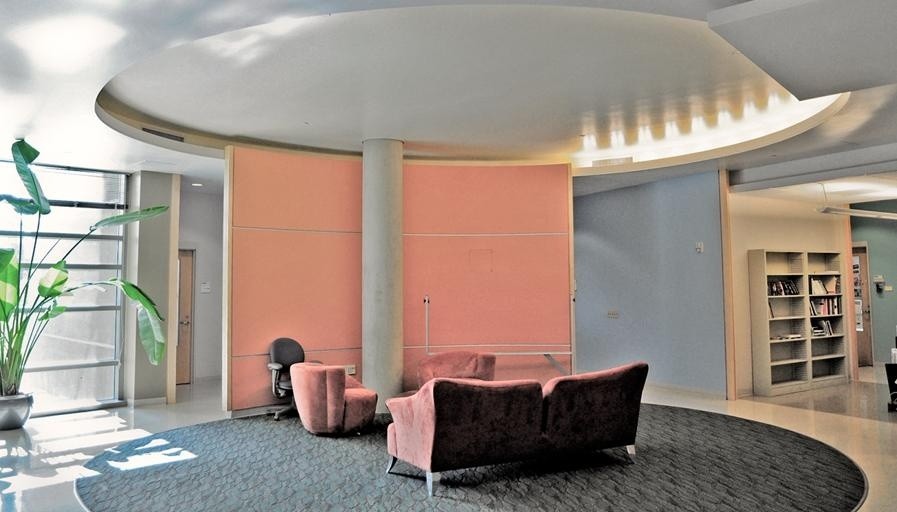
[73,398,869,511]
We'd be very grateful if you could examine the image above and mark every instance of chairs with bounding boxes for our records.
[543,361,649,469]
[385,377,543,494]
[290,360,379,437]
[398,350,497,396]
[265,338,305,421]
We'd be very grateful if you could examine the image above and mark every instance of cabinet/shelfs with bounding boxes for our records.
[751,247,848,397]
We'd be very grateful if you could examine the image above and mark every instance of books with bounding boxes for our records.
[767,276,841,340]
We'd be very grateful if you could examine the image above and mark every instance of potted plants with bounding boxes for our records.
[0,137,166,429]
